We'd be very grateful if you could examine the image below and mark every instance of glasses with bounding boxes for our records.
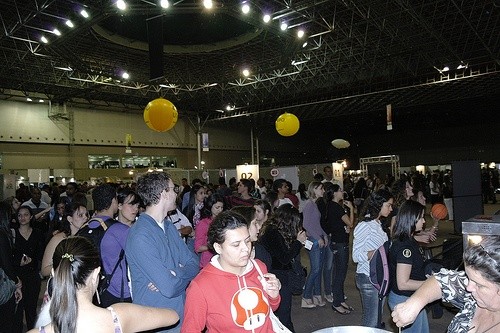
[163,187,179,193]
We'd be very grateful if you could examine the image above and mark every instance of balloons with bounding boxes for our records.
[144,99,178,133]
[276,113,300,136]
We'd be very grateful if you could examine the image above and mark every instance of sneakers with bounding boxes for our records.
[332,303,354,315]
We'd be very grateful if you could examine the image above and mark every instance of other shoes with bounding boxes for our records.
[313,295,327,306]
[324,294,333,303]
[331,292,347,299]
[301,297,316,308]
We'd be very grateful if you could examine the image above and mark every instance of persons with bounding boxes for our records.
[0,182,144,333]
[388,201,434,333]
[126,172,200,333]
[342,168,451,274]
[481,167,499,203]
[179,210,282,333]
[167,165,354,314]
[352,188,394,329]
[26,237,179,333]
[391,235,500,333]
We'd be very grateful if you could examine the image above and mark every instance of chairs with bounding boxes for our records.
[424,236,465,273]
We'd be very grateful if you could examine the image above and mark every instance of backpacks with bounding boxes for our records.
[74,218,130,301]
[369,239,396,300]
